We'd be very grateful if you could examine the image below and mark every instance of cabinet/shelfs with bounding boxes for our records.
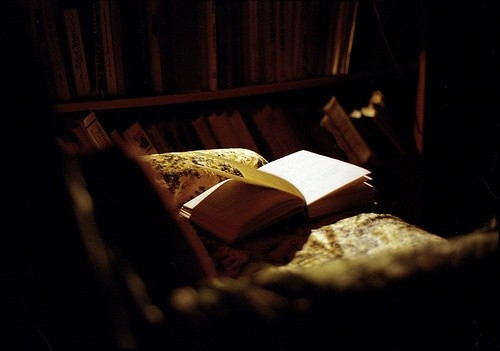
[0,0,432,228]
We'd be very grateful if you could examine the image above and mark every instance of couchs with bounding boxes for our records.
[0,146,499,351]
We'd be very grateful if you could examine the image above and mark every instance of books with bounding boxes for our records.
[178,149,376,249]
[25,3,382,190]
